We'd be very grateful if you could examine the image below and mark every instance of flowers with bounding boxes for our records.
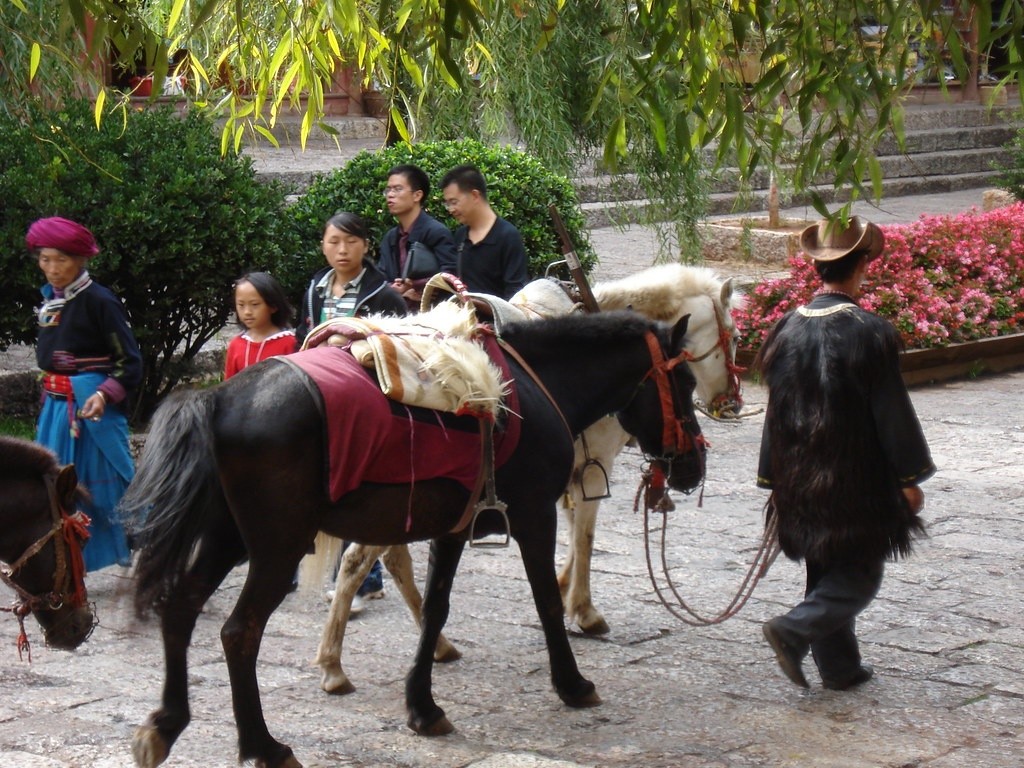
[729,201,1024,348]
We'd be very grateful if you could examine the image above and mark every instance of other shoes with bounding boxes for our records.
[326,586,384,602]
[762,623,810,689]
[822,666,873,690]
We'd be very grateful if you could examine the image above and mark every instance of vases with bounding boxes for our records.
[735,332,1024,390]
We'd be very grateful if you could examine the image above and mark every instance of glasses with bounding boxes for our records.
[443,191,471,211]
[383,188,416,196]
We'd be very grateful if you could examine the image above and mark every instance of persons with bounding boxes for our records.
[25,216,144,576]
[755,213,937,692]
[223,164,533,613]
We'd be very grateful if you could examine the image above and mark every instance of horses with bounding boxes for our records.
[97,262,741,768]
[0,436,94,650]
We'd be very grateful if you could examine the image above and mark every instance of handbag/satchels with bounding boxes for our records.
[400,241,439,285]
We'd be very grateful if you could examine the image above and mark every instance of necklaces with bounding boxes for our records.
[814,288,858,306]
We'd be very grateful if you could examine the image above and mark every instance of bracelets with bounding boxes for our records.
[96,391,106,406]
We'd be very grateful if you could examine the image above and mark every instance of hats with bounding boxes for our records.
[26,218,99,260]
[800,215,884,262]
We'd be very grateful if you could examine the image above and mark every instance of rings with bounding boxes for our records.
[94,416,101,423]
